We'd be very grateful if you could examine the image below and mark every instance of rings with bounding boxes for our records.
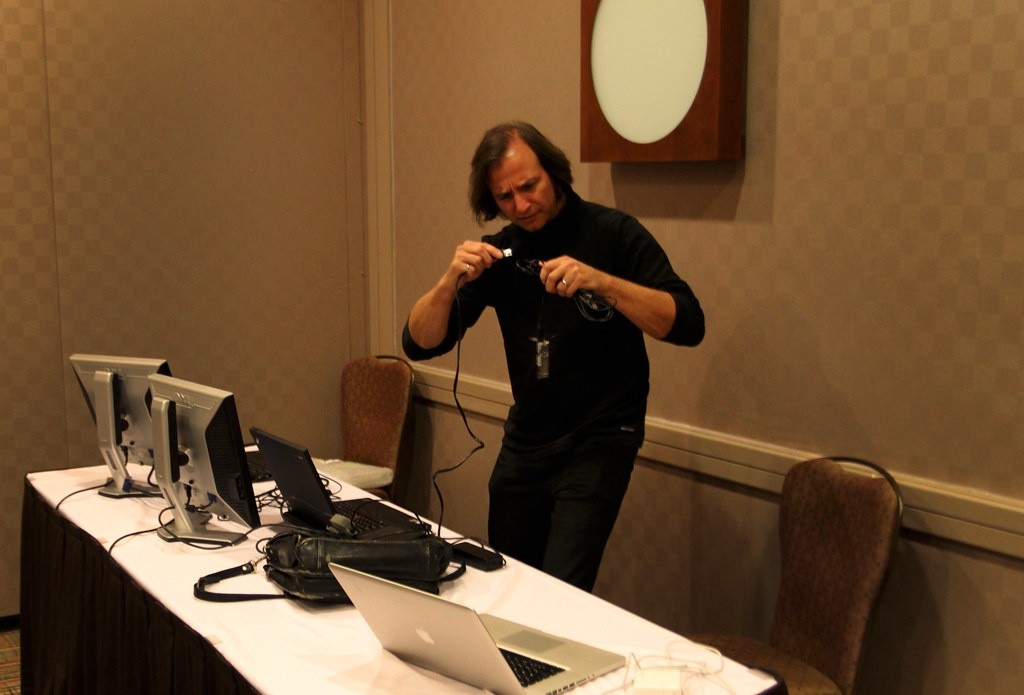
[562,279,567,287]
[467,264,470,270]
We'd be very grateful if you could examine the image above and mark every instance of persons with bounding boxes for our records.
[402,122,706,591]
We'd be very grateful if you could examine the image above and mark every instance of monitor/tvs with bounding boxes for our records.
[143,372,262,545]
[70,351,172,498]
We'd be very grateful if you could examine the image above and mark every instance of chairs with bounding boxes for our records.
[689,456,902,695]
[312,353,415,500]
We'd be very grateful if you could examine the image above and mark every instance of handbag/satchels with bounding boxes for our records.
[194,530,467,603]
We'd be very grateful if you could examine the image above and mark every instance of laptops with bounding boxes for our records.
[328,561,628,695]
[248,425,432,538]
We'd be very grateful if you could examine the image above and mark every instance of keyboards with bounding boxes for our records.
[242,452,275,481]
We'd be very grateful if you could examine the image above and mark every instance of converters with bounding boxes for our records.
[452,541,503,572]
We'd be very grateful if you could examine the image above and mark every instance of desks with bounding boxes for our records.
[22,445,788,695]
